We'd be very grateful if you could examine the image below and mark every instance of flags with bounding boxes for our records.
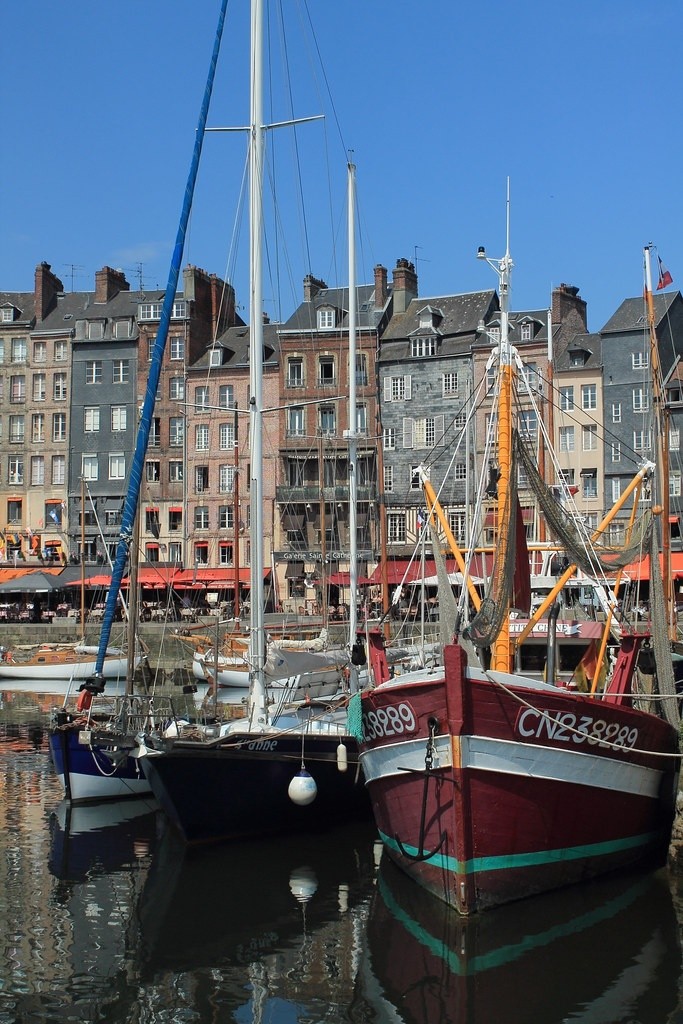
[417,508,425,528]
[657,256,673,290]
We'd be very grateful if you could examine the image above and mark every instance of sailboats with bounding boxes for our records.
[0,677,146,697]
[48,793,683,1024]
[0,0,683,913]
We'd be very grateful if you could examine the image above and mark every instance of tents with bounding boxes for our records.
[0,570,77,622]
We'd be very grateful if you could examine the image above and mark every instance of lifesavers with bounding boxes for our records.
[1,652,12,663]
[77,689,92,712]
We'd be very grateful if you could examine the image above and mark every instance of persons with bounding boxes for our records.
[389,585,428,622]
[180,594,192,608]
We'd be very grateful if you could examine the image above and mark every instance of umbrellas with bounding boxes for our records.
[311,571,490,597]
[66,575,241,608]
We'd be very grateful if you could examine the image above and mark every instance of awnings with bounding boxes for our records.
[584,553,683,582]
[68,564,272,589]
[284,562,306,580]
[371,553,543,584]
